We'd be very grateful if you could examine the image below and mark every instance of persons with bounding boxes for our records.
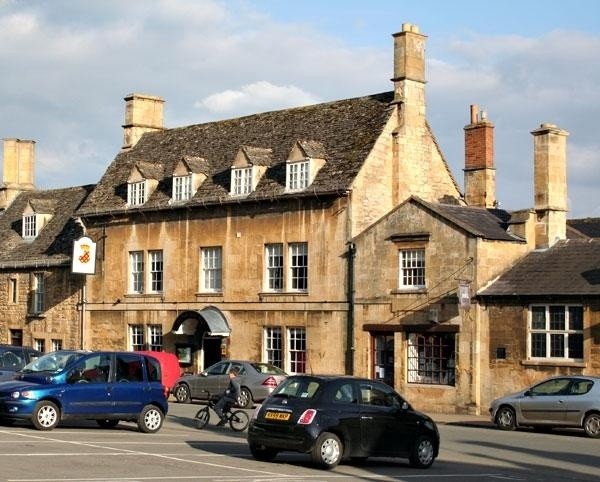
[212,368,243,429]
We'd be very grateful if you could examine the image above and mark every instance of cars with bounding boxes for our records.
[173,359,290,409]
[489,375,600,439]
[0,345,46,376]
[248,375,440,470]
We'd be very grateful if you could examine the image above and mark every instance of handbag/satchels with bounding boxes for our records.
[223,396,235,406]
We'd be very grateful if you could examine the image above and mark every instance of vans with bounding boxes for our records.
[0,349,168,433]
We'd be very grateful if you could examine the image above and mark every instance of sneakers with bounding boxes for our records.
[216,418,229,428]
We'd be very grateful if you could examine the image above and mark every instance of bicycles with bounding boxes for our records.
[194,389,249,432]
